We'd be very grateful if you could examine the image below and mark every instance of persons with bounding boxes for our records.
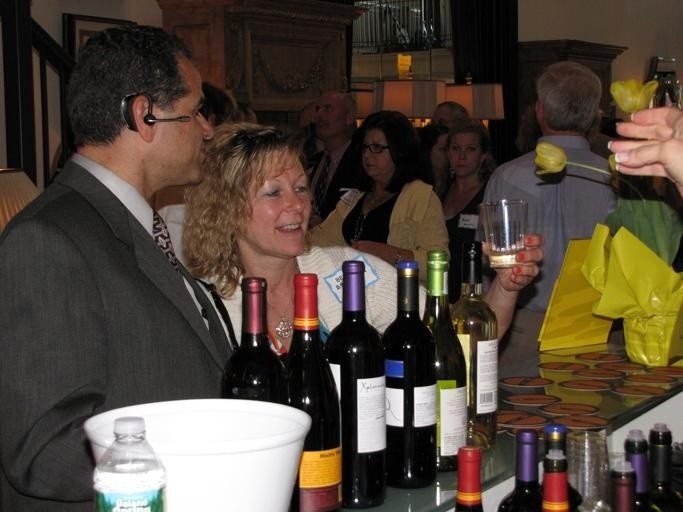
[180,121,544,356]
[0,23,239,512]
[474,61,617,314]
[152,79,495,301]
[608,106,683,198]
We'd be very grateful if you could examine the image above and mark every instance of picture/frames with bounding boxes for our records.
[61,13,139,172]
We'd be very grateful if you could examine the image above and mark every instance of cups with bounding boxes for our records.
[478,199,530,269]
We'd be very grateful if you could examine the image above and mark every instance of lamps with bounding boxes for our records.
[0,169,42,233]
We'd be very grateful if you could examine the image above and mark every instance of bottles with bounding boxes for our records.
[421,250,468,473]
[324,259,387,510]
[286,272,343,512]
[220,275,288,406]
[455,423,683,512]
[91,416,167,512]
[382,260,439,489]
[448,240,499,451]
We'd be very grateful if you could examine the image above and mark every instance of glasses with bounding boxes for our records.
[360,143,391,155]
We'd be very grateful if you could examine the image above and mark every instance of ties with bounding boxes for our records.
[153,210,182,283]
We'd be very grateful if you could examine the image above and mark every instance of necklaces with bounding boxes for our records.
[265,297,293,340]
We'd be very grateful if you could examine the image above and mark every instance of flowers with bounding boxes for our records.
[532,78,682,267]
